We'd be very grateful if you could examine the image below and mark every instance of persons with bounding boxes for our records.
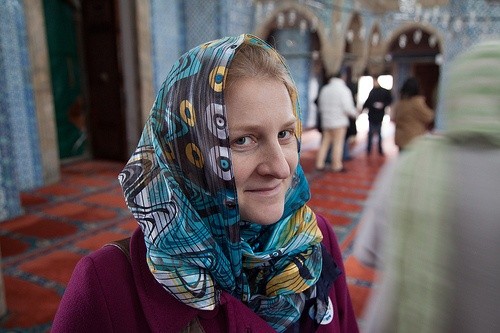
[353,39,500,332]
[312,65,437,172]
[49,36,361,333]
